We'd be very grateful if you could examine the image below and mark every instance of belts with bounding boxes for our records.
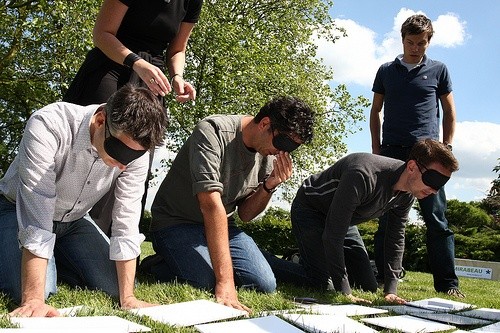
[379,144,413,150]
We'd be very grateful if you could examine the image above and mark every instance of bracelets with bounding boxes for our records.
[123,51,142,71]
[170,73,184,89]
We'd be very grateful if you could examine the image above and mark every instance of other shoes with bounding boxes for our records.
[139,252,164,273]
[435,287,466,299]
[291,253,300,265]
[376,271,385,290]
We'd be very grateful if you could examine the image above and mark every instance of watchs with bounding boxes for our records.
[262,178,278,196]
[444,141,453,151]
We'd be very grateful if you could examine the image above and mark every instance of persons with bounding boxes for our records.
[258,140,461,305]
[137,94,317,317]
[368,13,468,302]
[0,82,169,318]
[63,0,204,240]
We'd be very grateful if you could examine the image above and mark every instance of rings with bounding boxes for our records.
[148,78,155,84]
